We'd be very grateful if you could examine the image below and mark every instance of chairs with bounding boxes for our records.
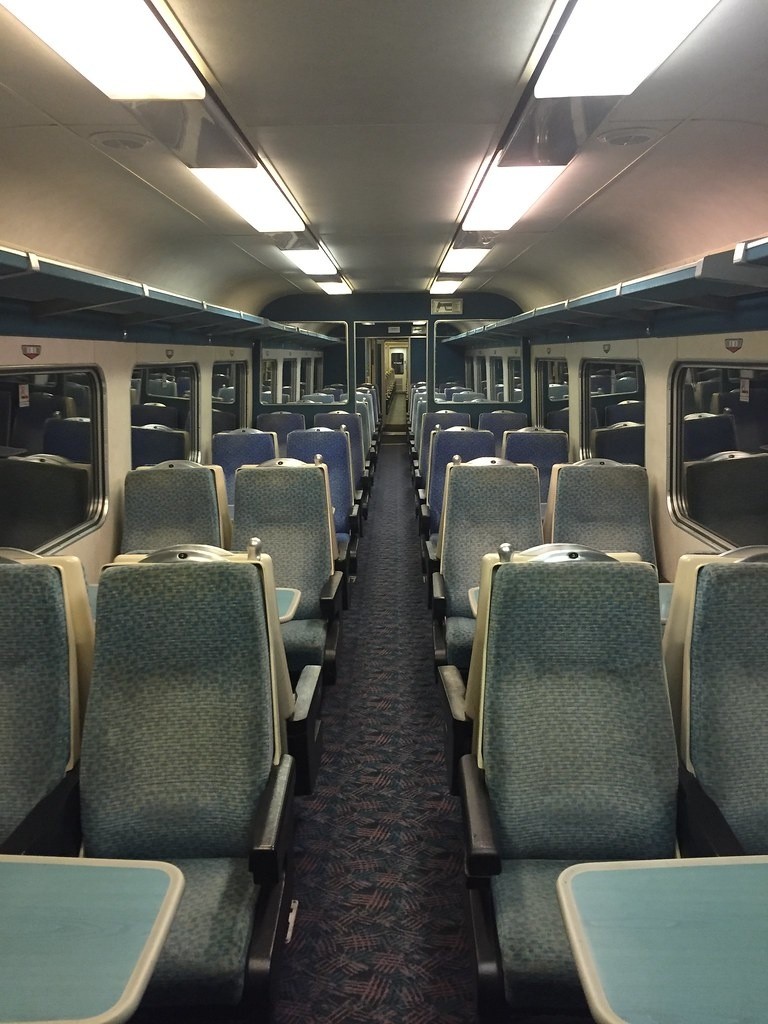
[0,367,768,1023]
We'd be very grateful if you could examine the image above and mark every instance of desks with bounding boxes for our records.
[226,504,336,524]
[555,854,768,1024]
[468,583,675,625]
[85,583,302,624]
[0,853,185,1024]
